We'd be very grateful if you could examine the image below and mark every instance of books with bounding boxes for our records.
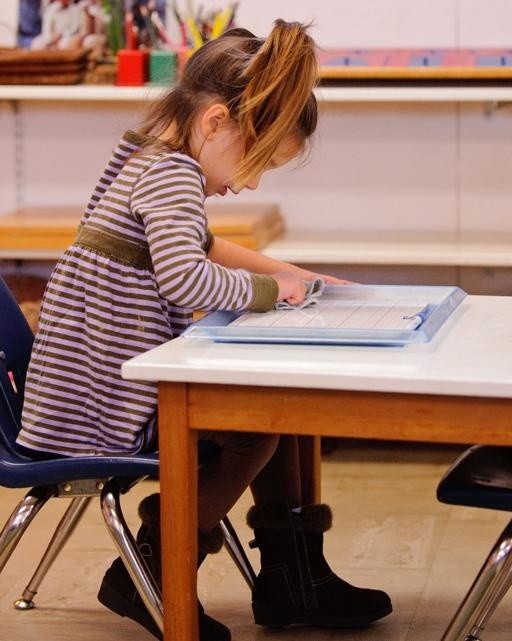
[215,272,469,344]
[0,45,90,85]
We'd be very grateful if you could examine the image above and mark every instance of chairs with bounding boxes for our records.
[437,443,512,640]
[2,282,255,641]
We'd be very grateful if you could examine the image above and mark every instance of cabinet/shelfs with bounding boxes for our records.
[1,83,511,270]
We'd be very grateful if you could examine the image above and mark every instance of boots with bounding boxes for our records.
[246,505,393,627]
[97,494,231,641]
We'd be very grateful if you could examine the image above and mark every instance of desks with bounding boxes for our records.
[121,296,512,639]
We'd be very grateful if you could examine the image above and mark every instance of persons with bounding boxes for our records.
[22,17,395,638]
